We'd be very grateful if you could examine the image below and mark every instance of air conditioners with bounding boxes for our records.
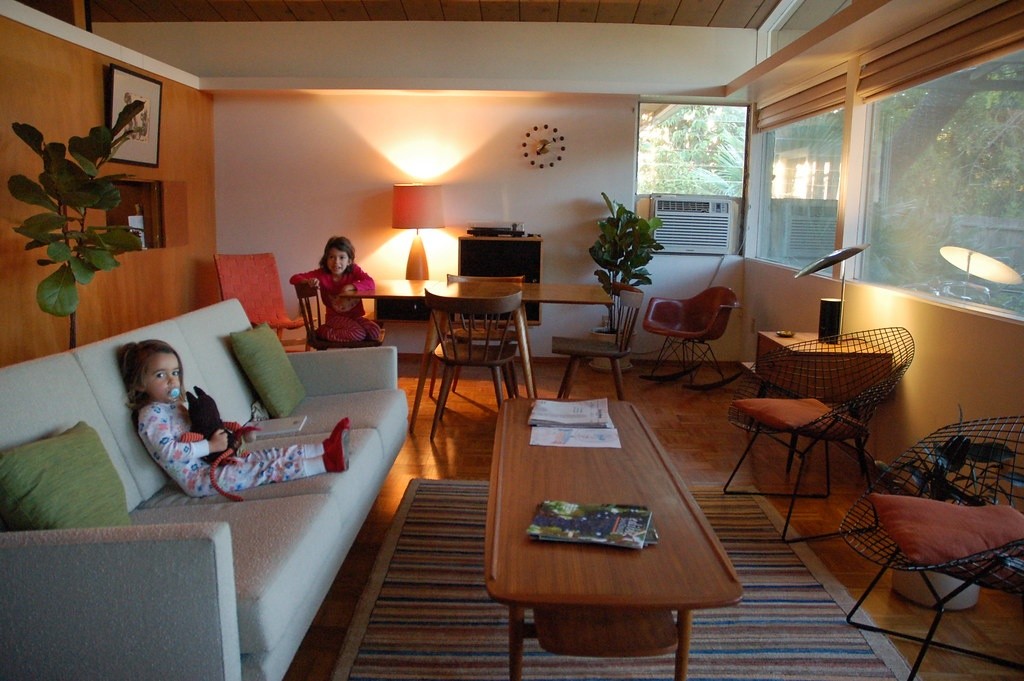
[648,193,732,254]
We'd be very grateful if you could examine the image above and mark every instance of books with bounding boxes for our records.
[524,499,661,551]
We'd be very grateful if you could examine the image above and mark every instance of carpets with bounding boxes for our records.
[331,477,927,680]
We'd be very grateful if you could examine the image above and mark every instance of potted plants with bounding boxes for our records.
[589,191,664,374]
[856,437,1024,608]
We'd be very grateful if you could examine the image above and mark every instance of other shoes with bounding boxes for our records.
[323,429,349,473]
[321,417,350,451]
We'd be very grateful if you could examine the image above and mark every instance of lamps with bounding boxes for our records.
[938,244,1022,305]
[793,241,871,343]
[391,184,446,280]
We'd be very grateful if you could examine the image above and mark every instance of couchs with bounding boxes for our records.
[0,298,411,681]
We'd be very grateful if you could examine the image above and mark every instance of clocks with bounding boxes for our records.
[522,124,565,169]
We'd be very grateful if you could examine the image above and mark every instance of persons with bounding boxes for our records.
[120,339,352,499]
[287,235,380,341]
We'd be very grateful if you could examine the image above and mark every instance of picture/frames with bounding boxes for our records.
[106,62,162,167]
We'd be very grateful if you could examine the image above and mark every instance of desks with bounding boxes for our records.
[338,279,615,431]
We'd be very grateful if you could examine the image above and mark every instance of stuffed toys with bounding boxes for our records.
[179,385,262,502]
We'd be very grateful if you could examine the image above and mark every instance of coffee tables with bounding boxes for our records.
[485,398,743,680]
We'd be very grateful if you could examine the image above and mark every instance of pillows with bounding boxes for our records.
[1,322,305,530]
[871,492,1024,565]
[731,391,865,437]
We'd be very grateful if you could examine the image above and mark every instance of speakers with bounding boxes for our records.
[456,233,543,326]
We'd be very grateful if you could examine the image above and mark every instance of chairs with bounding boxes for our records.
[723,328,914,541]
[552,283,644,401]
[213,251,317,352]
[425,275,522,440]
[294,284,384,351]
[838,417,1024,681]
[638,285,744,391]
